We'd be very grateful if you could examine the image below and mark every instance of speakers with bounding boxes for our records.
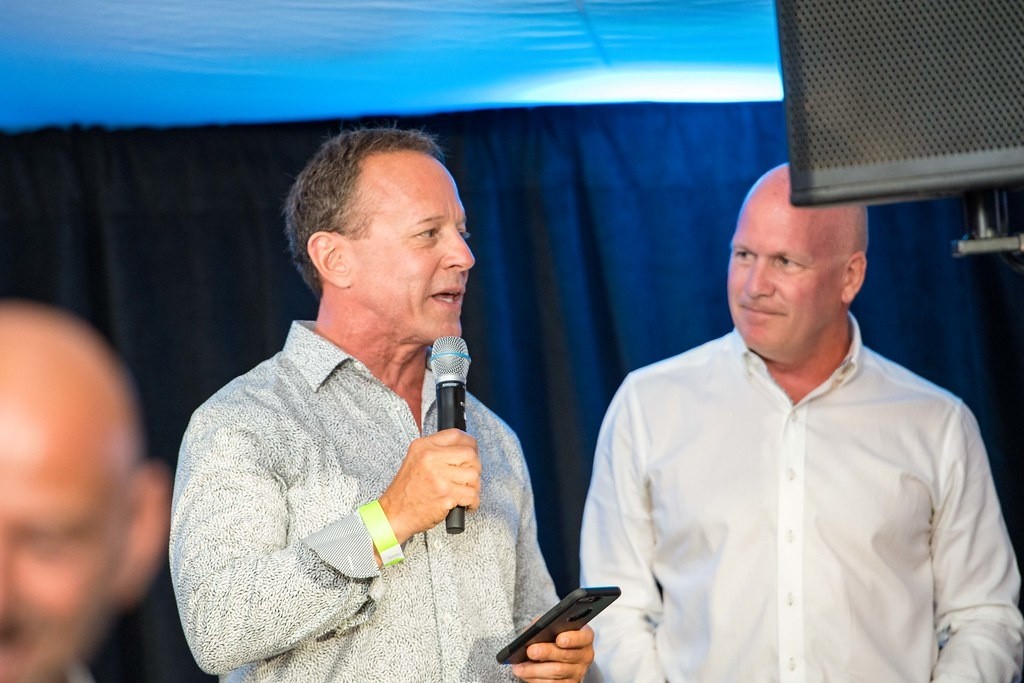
[776,0,1024,210]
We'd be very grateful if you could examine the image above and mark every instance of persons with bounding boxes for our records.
[169,128,623,683]
[0,303,171,683]
[579,164,1024,683]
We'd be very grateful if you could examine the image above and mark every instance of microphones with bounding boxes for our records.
[428,335,471,533]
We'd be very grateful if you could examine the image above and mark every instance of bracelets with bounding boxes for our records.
[359,500,404,568]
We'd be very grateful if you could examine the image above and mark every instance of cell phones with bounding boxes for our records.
[496,587,621,665]
